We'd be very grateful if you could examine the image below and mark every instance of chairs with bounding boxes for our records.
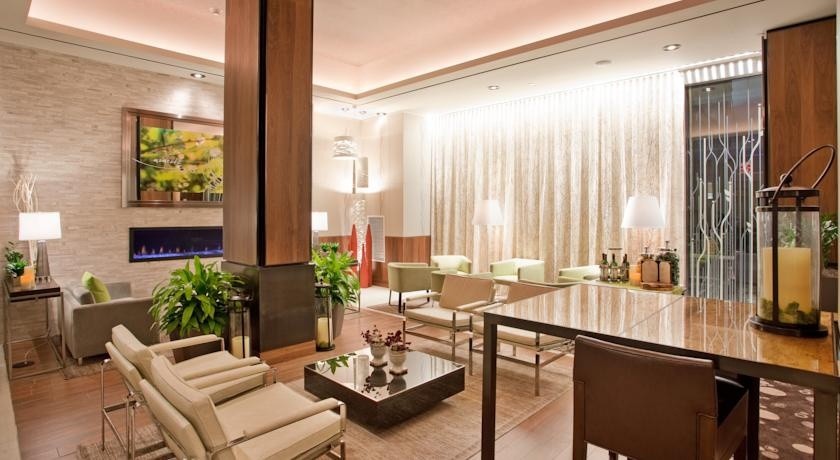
[99,324,347,460]
[490,258,544,283]
[559,264,600,285]
[572,334,747,460]
[402,272,574,395]
[387,262,440,313]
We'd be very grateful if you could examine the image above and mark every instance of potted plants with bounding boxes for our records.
[147,256,254,364]
[311,242,364,340]
[5,250,24,286]
[384,330,416,366]
[360,324,386,360]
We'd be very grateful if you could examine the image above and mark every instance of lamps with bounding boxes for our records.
[312,211,328,244]
[19,211,63,283]
[619,195,663,254]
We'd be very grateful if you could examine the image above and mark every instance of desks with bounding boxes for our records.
[480,284,840,460]
[596,278,683,295]
[304,346,466,429]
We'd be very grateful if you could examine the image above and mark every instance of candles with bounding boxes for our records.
[318,317,333,344]
[763,247,813,312]
[232,336,250,358]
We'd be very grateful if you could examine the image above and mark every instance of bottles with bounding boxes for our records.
[600,253,629,282]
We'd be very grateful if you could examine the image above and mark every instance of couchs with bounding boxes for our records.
[61,281,161,366]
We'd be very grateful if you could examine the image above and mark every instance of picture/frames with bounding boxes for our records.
[122,107,224,208]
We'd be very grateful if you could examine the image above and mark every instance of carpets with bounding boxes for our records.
[75,348,572,460]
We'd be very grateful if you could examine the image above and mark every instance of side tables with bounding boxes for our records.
[3,278,66,382]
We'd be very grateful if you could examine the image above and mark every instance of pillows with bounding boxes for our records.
[81,273,111,304]
